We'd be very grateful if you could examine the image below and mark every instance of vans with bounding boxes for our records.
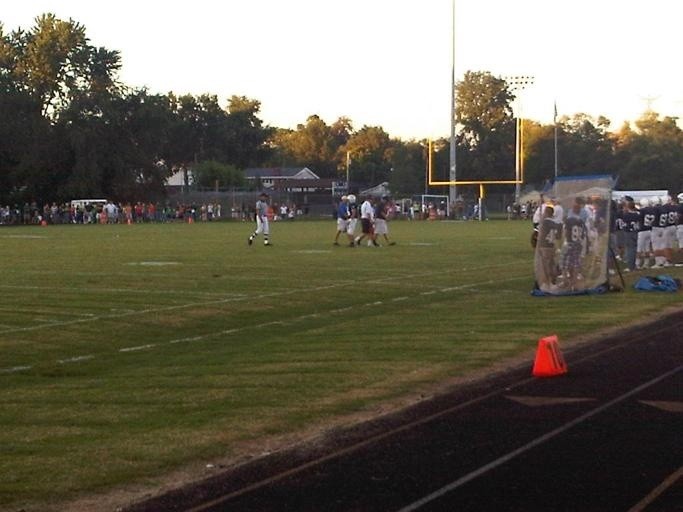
[70,199,119,224]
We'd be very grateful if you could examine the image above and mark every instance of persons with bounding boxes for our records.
[394,203,400,216]
[354,194,377,248]
[273,202,295,220]
[246,192,273,247]
[1,200,221,226]
[472,202,478,216]
[504,190,682,294]
[332,195,348,246]
[373,193,396,247]
[230,204,247,222]
[345,194,358,248]
[413,200,446,222]
[408,203,416,225]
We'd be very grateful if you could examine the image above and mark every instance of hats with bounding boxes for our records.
[341,195,347,200]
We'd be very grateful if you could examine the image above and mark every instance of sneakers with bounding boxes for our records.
[557,262,683,281]
[246,237,274,247]
[333,240,396,249]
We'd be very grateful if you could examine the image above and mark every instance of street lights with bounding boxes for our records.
[501,73,538,204]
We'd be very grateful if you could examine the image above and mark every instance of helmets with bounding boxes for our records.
[639,195,671,207]
[346,194,357,204]
[677,193,683,204]
